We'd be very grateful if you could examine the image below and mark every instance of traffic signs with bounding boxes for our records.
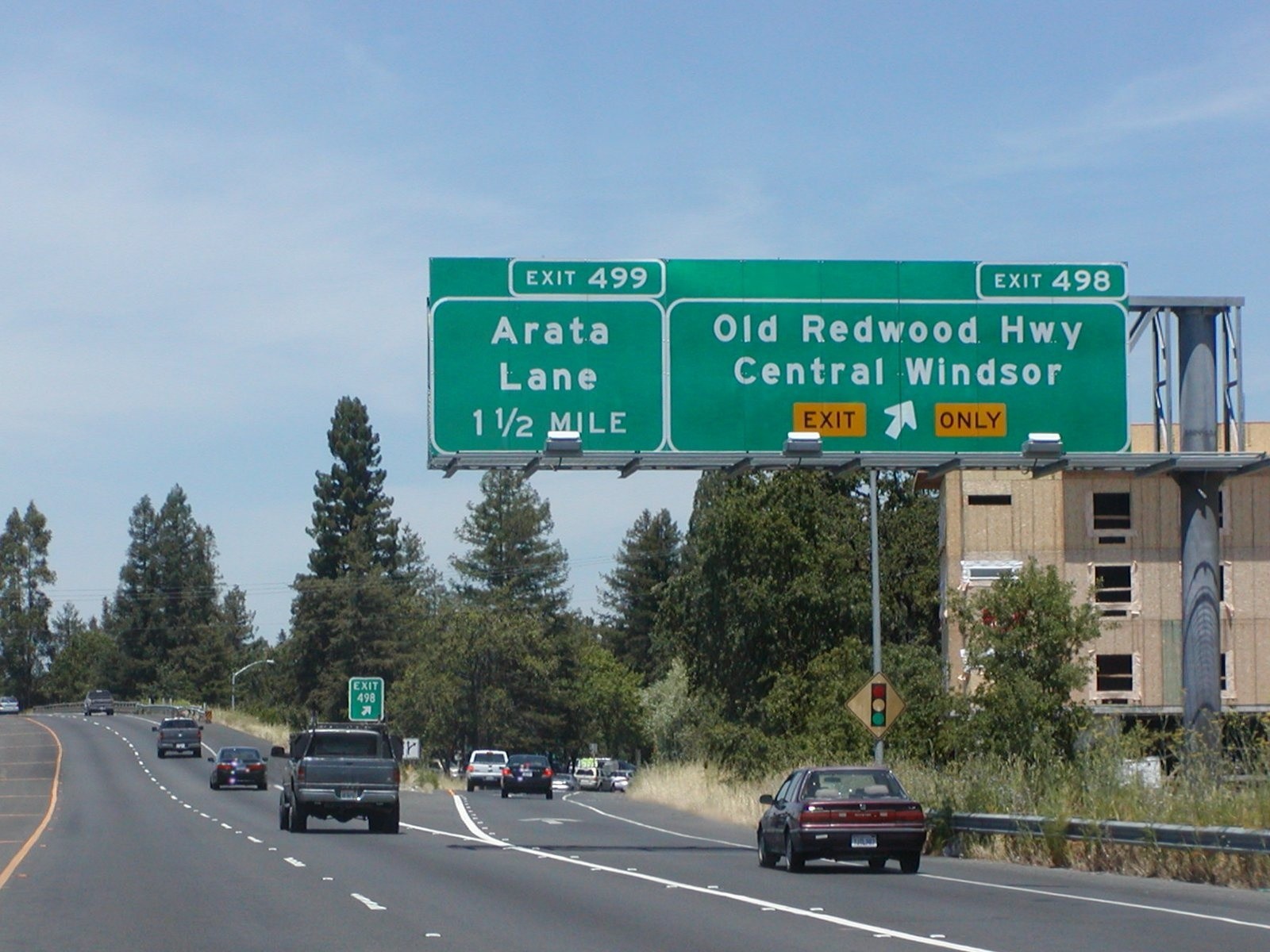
[427,254,1130,455]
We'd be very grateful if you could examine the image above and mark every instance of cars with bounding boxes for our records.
[83,688,114,716]
[463,748,508,787]
[754,765,928,875]
[206,746,270,791]
[152,716,206,757]
[552,757,637,794]
[500,752,554,800]
[0,696,20,714]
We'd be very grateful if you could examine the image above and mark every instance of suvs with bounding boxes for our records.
[265,720,403,834]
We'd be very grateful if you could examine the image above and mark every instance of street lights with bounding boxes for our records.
[229,658,276,711]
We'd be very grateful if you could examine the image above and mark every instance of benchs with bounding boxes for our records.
[814,785,889,800]
